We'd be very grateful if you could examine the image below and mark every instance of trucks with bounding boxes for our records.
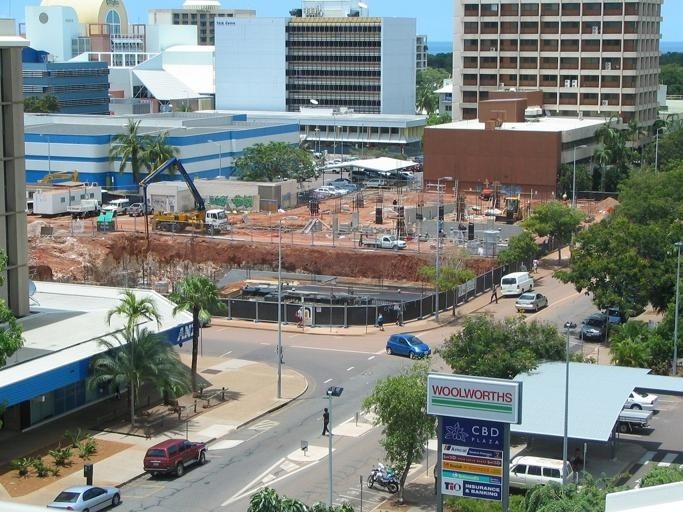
[616,407,654,433]
[66,198,101,220]
[362,234,407,251]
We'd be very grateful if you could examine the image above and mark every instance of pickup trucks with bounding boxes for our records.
[126,202,154,217]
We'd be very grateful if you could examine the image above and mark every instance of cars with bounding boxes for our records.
[46,483,122,512]
[313,158,414,199]
[623,387,659,411]
[579,305,623,342]
[514,292,549,312]
[385,333,432,360]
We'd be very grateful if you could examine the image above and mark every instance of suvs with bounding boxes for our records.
[143,438,207,478]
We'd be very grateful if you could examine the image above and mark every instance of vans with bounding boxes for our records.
[508,455,575,497]
[102,198,130,217]
[500,270,534,297]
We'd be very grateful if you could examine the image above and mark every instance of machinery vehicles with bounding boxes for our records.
[493,195,524,223]
[138,157,229,253]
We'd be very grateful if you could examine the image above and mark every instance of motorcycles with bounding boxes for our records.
[366,458,399,494]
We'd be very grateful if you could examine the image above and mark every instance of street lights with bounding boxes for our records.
[435,176,454,322]
[275,214,299,398]
[655,126,667,170]
[562,321,578,487]
[326,386,344,508]
[572,144,587,210]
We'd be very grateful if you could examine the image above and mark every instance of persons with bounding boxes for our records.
[377,312,385,331]
[296,306,304,327]
[489,283,498,304]
[532,258,539,273]
[519,263,528,272]
[321,408,329,435]
